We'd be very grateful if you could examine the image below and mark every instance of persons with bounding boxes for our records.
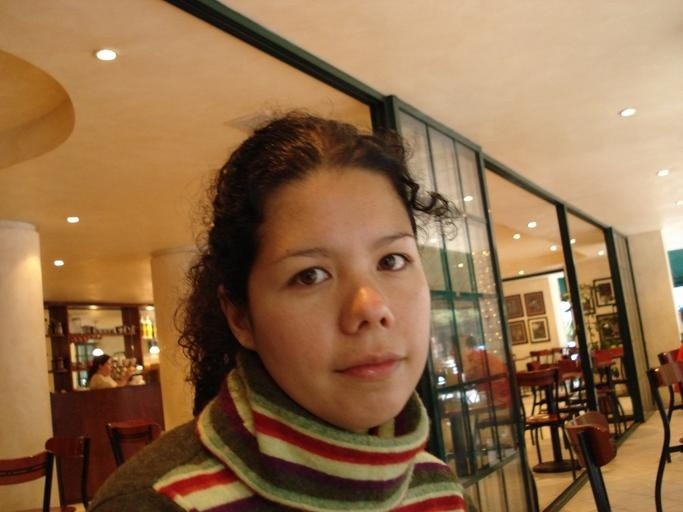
[89,355,134,388]
[450,336,511,420]
[90,109,466,510]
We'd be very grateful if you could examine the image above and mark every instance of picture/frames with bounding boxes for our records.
[597,313,623,350]
[591,276,614,308]
[502,289,551,346]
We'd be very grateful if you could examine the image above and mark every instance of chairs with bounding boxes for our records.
[105,421,162,465]
[0,450,55,512]
[43,432,101,512]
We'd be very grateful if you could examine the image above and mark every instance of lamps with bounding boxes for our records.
[148,340,159,353]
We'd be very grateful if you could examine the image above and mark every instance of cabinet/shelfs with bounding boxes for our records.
[44,300,142,391]
